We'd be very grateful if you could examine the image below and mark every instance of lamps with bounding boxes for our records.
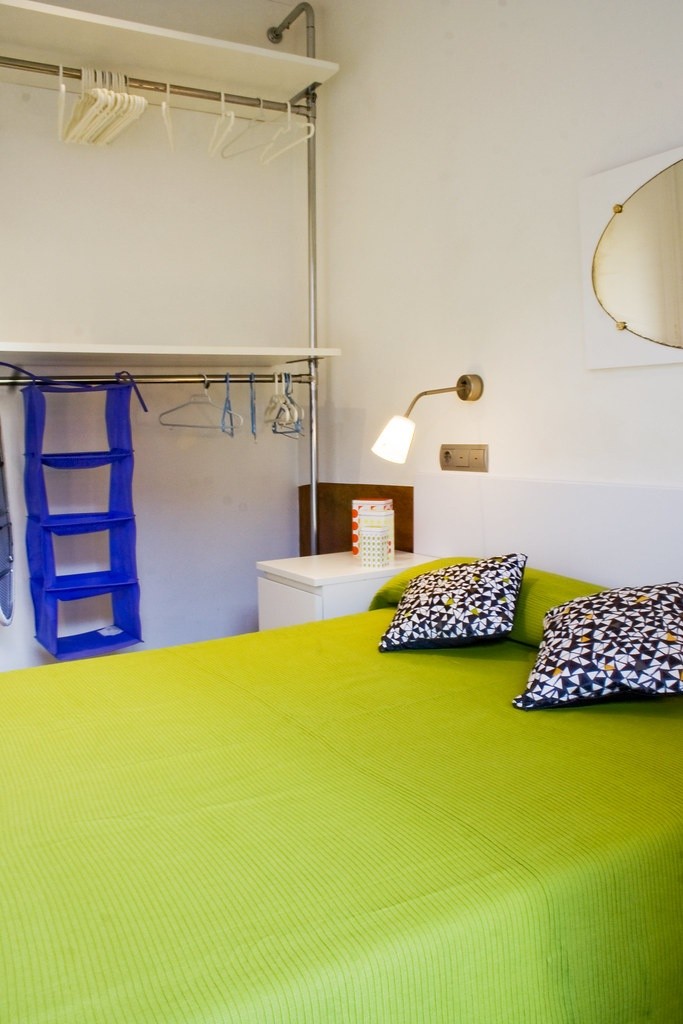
[369,374,485,466]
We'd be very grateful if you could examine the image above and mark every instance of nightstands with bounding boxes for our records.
[255,544,442,631]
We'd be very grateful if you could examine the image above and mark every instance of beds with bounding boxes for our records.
[0,552,683,1024]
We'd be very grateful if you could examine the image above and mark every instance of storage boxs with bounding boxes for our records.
[352,497,395,569]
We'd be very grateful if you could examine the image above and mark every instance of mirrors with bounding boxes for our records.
[591,158,683,350]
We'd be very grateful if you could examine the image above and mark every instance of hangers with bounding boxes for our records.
[159,372,308,440]
[57,62,315,165]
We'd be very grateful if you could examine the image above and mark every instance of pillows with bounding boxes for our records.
[512,580,683,712]
[378,552,528,652]
